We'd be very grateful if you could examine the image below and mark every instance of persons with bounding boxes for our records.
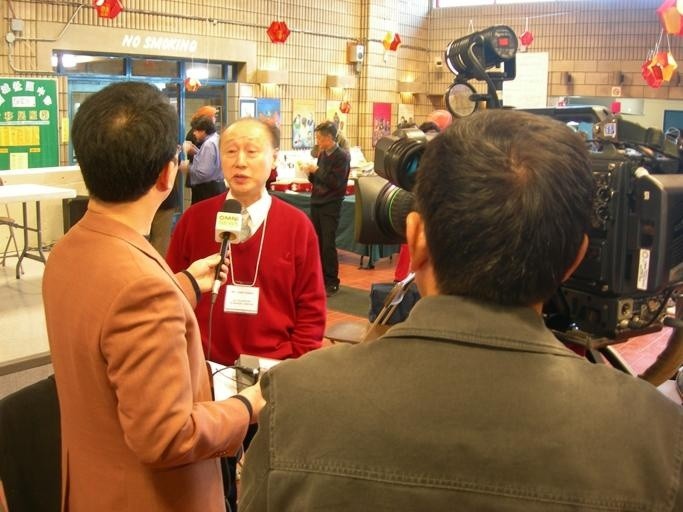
[167,119,328,512]
[665,127,680,140]
[395,111,452,281]
[240,110,682,512]
[300,121,351,296]
[151,106,226,258]
[42,82,265,512]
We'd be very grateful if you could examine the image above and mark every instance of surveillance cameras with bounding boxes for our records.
[434,57,443,67]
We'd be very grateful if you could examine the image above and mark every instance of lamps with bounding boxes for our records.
[327,74,356,89]
[641,0,683,88]
[519,31,534,46]
[382,31,401,52]
[184,58,202,93]
[94,0,124,19]
[398,80,424,93]
[256,68,290,86]
[266,21,291,43]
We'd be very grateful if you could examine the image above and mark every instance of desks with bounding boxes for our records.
[0,182,77,280]
[267,189,401,271]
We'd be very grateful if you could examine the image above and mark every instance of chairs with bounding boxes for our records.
[0,177,25,274]
[0,373,62,512]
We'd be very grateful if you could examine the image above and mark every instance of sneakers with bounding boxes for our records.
[325,284,339,296]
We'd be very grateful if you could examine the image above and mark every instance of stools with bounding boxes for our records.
[324,321,366,344]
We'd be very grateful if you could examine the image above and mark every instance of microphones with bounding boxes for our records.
[210,199,242,304]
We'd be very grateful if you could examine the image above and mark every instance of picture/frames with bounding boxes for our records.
[239,98,257,119]
